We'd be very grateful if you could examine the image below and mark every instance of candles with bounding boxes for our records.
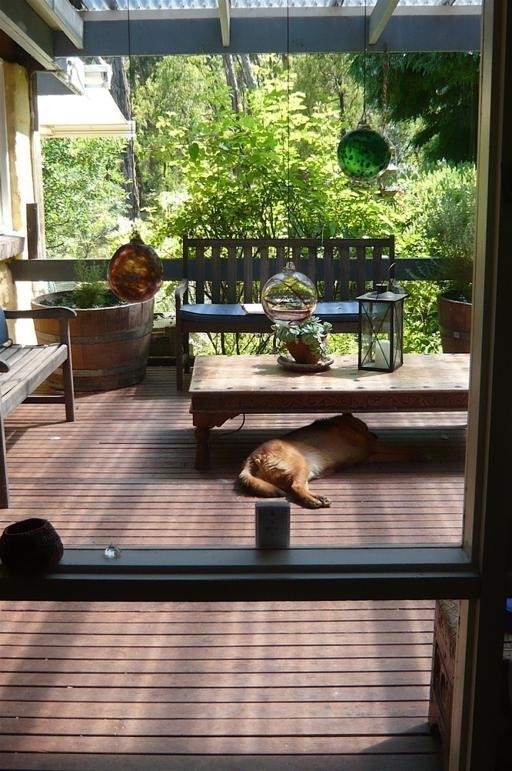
[375,338,390,369]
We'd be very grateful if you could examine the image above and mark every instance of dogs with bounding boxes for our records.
[236,413,434,508]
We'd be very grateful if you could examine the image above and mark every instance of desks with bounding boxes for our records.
[189,351,469,470]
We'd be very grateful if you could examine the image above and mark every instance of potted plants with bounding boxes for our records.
[270,310,334,364]
[32,240,154,391]
[424,183,476,352]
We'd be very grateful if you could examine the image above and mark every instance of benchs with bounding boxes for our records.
[171,232,396,389]
[1,305,80,513]
